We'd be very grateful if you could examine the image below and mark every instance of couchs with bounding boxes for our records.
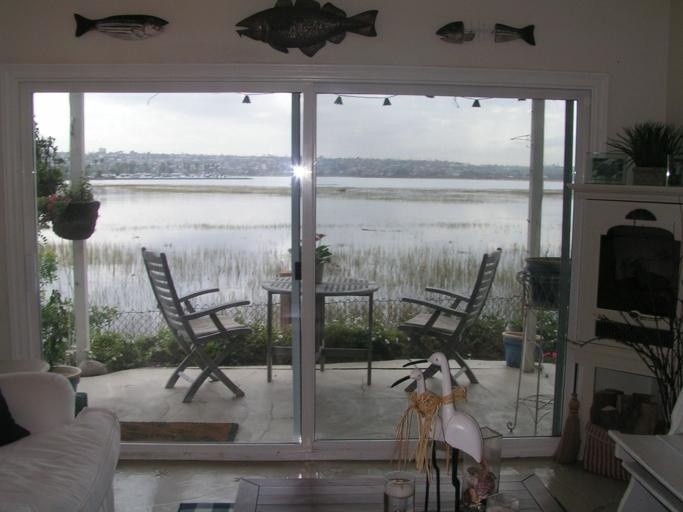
[1,368,126,511]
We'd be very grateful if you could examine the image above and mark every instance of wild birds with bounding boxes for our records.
[391,368,446,512]
[402,351,488,512]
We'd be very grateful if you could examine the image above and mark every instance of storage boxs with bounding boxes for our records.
[580,418,659,483]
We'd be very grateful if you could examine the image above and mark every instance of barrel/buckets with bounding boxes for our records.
[524,256,570,308]
[503,330,545,367]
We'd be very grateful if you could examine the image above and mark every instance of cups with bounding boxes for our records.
[483,494,519,512]
[383,479,416,512]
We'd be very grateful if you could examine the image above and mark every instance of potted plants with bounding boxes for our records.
[604,120,683,186]
[285,228,333,284]
[46,174,102,243]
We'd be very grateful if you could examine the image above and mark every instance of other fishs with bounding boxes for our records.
[434,22,537,47]
[74,12,169,42]
[236,0,379,59]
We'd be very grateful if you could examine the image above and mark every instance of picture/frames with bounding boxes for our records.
[583,151,629,184]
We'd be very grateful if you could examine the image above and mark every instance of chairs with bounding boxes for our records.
[392,246,508,394]
[141,244,257,405]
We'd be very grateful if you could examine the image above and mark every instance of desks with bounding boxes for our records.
[261,274,383,388]
[604,424,682,510]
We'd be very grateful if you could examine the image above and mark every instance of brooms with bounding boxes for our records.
[549,362,582,473]
